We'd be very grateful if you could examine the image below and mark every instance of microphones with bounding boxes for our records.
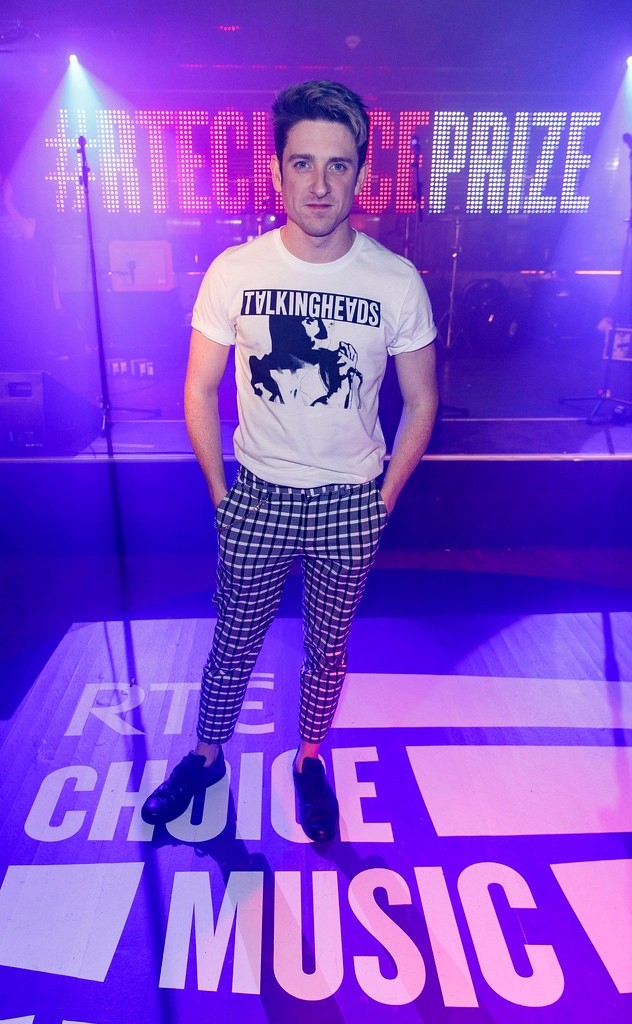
[412,135,421,153]
[623,134,632,148]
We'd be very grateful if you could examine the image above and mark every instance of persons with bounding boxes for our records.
[0,151,57,372]
[138,79,440,844]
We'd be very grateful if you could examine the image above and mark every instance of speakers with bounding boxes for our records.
[0,369,102,455]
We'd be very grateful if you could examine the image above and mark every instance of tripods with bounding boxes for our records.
[559,206,632,424]
[438,204,473,354]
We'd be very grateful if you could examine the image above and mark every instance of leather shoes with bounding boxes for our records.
[141,745,226,825]
[292,745,339,842]
